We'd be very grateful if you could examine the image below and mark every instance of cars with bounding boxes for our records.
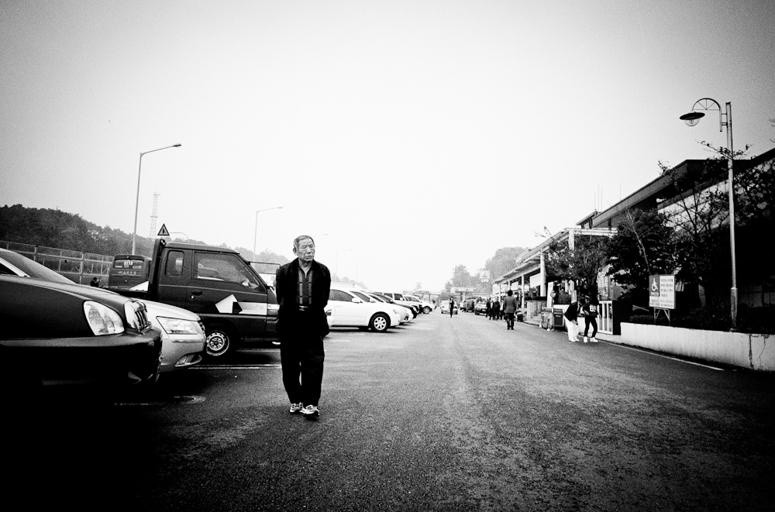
[459,296,491,316]
[0,275,163,424]
[440,300,459,315]
[0,244,211,382]
[256,273,435,331]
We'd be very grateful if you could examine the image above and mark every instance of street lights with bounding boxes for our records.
[252,206,283,266]
[679,96,743,333]
[131,141,182,256]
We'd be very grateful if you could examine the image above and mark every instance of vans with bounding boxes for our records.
[107,255,151,290]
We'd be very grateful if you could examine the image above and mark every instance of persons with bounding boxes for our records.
[564,298,588,342]
[582,292,601,343]
[487,296,506,320]
[274,234,331,419]
[504,290,518,330]
[448,296,454,318]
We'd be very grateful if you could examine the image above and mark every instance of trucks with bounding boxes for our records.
[84,240,282,362]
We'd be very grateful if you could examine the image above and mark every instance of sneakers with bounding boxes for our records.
[299,405,319,418]
[290,402,300,414]
